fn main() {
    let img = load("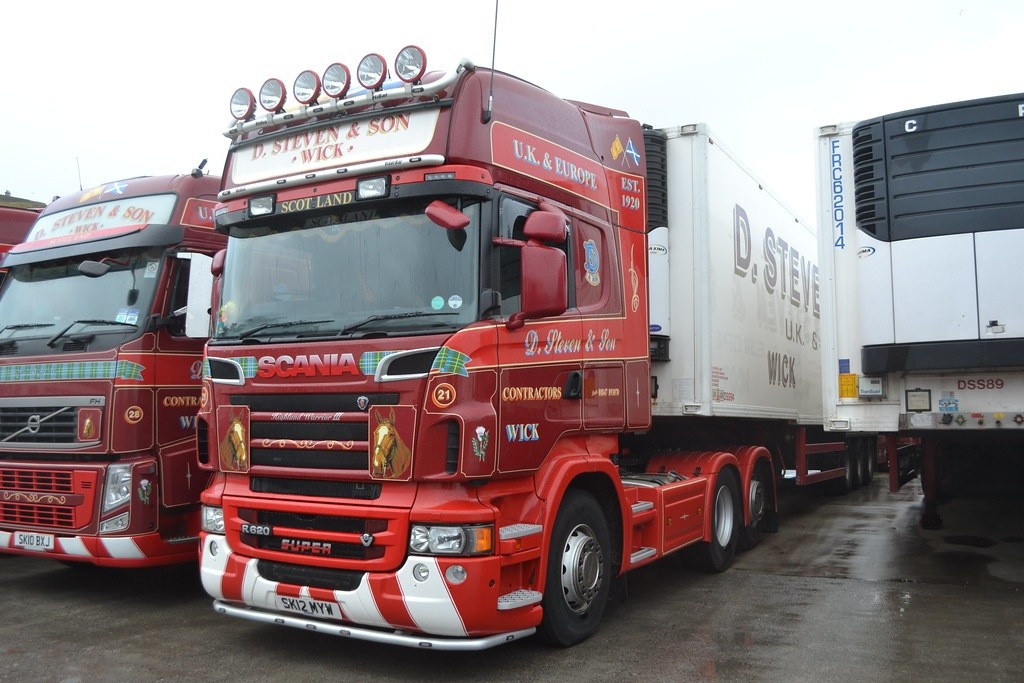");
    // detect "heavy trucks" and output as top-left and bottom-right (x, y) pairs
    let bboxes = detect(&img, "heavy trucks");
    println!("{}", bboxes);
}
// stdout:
(1, 155), (224, 573)
(191, 0), (877, 655)
(813, 92), (1024, 514)
